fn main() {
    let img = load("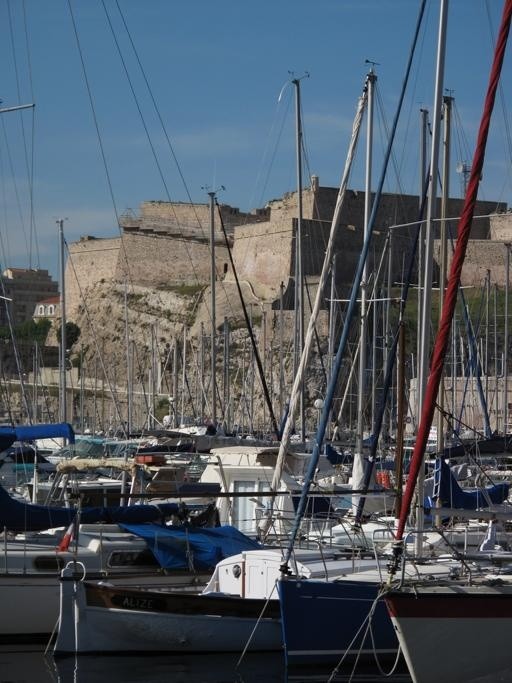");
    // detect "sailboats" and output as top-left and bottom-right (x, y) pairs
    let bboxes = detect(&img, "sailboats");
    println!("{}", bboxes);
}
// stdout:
(1, 0), (512, 682)
